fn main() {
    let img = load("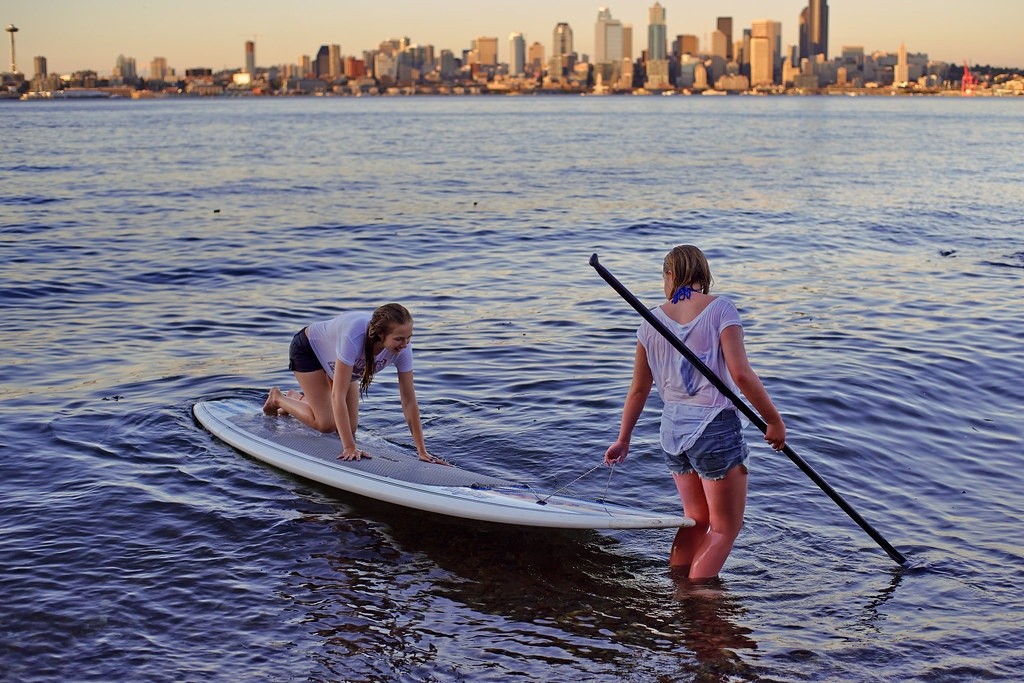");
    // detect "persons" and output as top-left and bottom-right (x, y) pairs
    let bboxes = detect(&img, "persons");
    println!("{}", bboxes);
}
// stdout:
(262, 303), (450, 465)
(605, 244), (786, 580)
